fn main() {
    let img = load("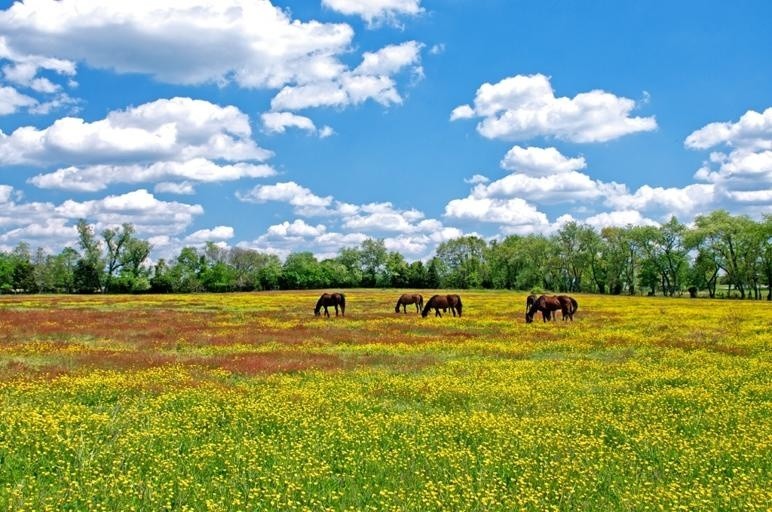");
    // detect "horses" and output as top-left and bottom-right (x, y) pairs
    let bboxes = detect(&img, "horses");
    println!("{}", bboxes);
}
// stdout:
(422, 294), (463, 318)
(525, 294), (578, 323)
(314, 293), (345, 318)
(395, 294), (423, 314)
